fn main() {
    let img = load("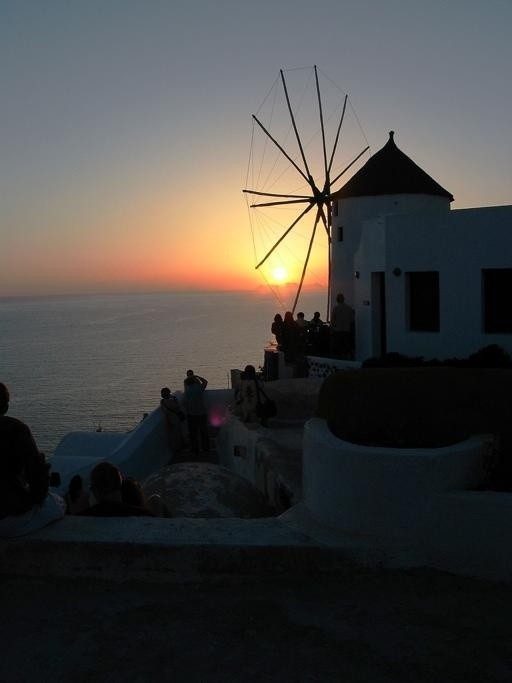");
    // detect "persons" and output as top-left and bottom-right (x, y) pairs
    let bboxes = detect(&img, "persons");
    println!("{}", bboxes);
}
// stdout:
(242, 364), (273, 428)
(272, 312), (283, 335)
(161, 387), (187, 453)
(45, 458), (163, 518)
(1, 382), (68, 537)
(182, 375), (211, 455)
(293, 311), (310, 327)
(282, 310), (297, 330)
(183, 369), (200, 391)
(308, 311), (324, 326)
(329, 292), (354, 354)
